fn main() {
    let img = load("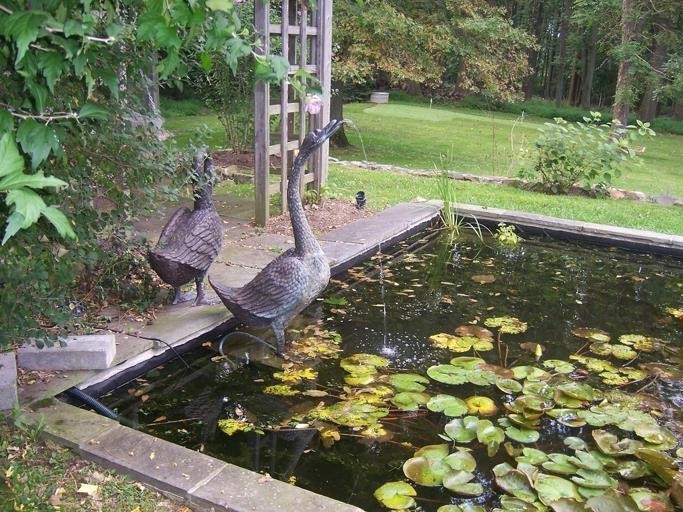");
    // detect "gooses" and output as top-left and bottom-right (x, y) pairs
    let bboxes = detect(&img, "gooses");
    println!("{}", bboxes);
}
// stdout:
(207, 119), (341, 355)
(147, 147), (224, 307)
